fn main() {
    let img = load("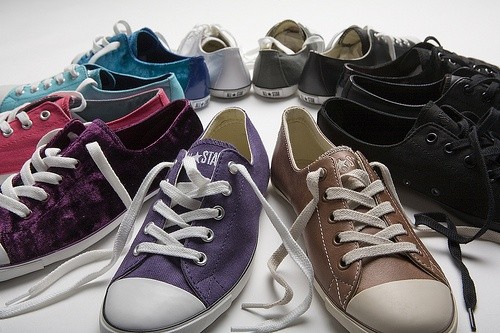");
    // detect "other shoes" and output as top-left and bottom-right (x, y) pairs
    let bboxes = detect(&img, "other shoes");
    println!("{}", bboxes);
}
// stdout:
(0, 108), (315, 333)
(176, 22), (251, 101)
(244, 21), (326, 99)
(297, 26), (424, 104)
(70, 21), (212, 108)
(0, 97), (205, 307)
(317, 95), (500, 332)
(1, 63), (183, 123)
(0, 89), (171, 185)
(340, 72), (500, 140)
(230, 105), (459, 333)
(336, 36), (499, 99)
